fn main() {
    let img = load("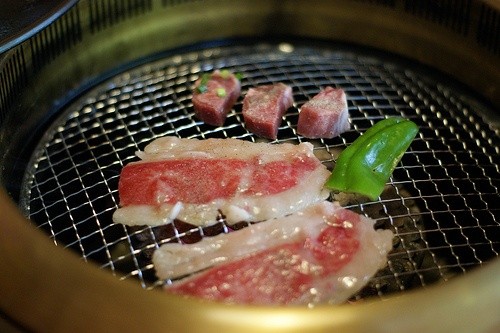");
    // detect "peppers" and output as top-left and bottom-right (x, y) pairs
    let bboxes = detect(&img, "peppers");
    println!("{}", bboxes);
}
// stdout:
(323, 114), (420, 202)
